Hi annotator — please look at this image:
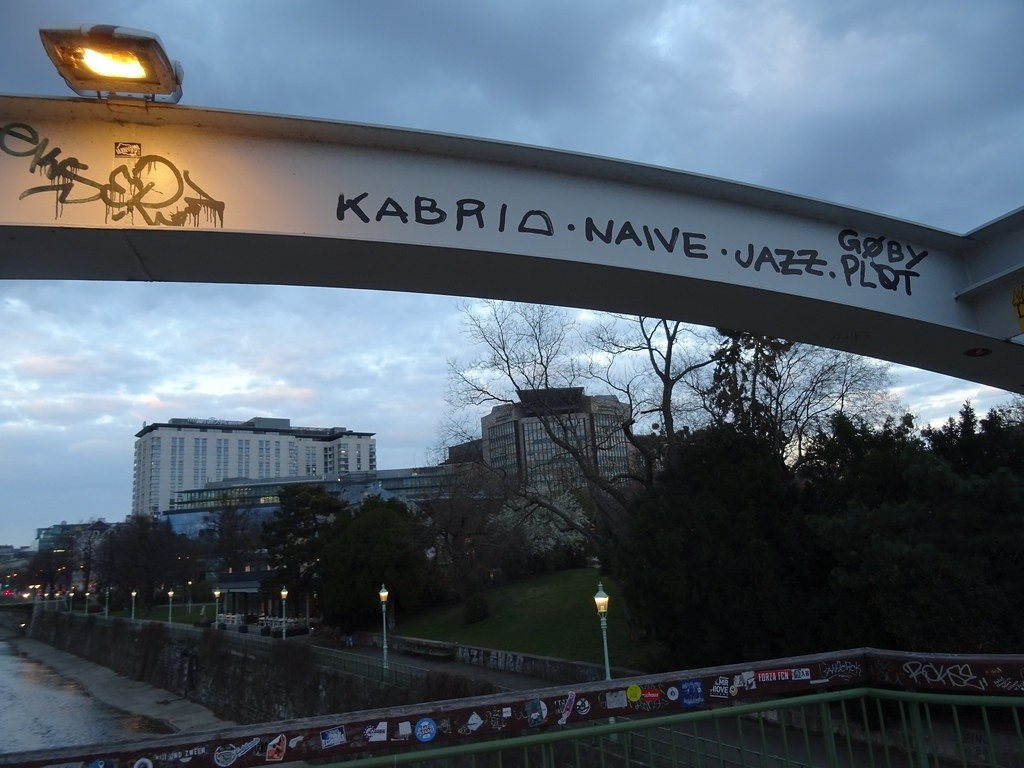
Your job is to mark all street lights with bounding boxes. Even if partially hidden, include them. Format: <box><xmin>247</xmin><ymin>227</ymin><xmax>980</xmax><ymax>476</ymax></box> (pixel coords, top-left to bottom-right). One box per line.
<box><xmin>280</xmin><ymin>585</ymin><xmax>288</xmax><ymax>640</ymax></box>
<box><xmin>105</xmin><ymin>591</ymin><xmax>109</xmax><ymax>617</ymax></box>
<box><xmin>168</xmin><ymin>588</ymin><xmax>175</xmax><ymax>624</ymax></box>
<box><xmin>187</xmin><ymin>579</ymin><xmax>192</xmax><ymax>614</ymax></box>
<box><xmin>68</xmin><ymin>592</ymin><xmax>74</xmax><ymax>613</ymax></box>
<box><xmin>84</xmin><ymin>592</ymin><xmax>90</xmax><ymax>614</ymax></box>
<box><xmin>378</xmin><ymin>583</ymin><xmax>389</xmax><ymax>668</ymax></box>
<box><xmin>213</xmin><ymin>586</ymin><xmax>220</xmax><ymax>629</ymax></box>
<box><xmin>594</xmin><ymin>581</ymin><xmax>611</xmax><ymax>680</ymax></box>
<box><xmin>131</xmin><ymin>589</ymin><xmax>137</xmax><ymax>620</ymax></box>
